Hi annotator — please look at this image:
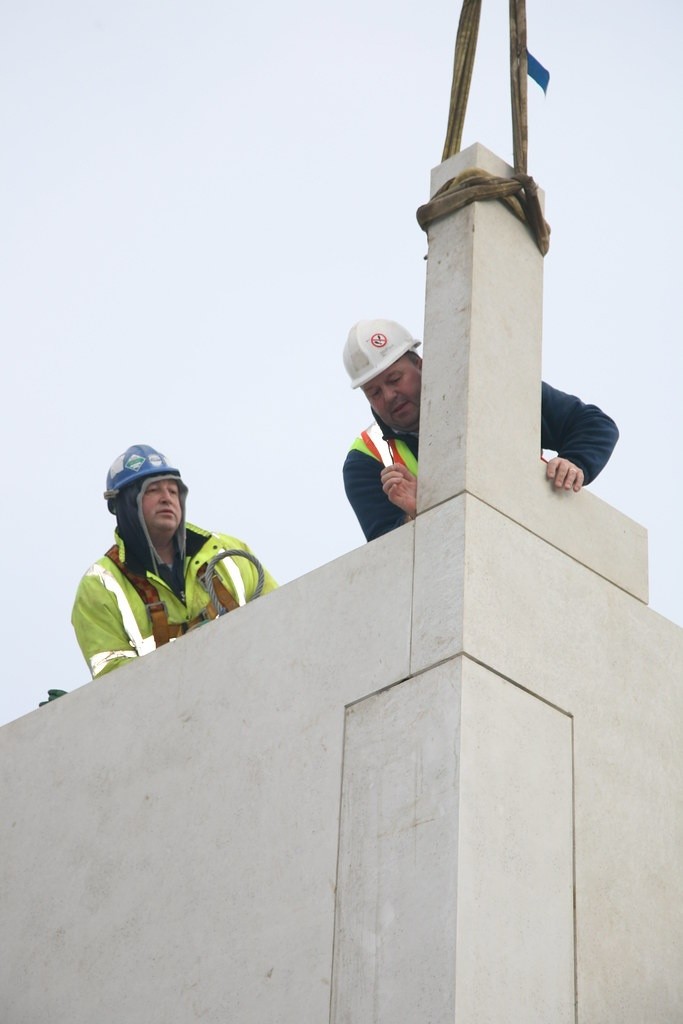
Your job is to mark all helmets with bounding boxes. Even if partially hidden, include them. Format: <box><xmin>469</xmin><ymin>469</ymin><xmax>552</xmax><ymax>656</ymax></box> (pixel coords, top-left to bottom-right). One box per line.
<box><xmin>102</xmin><ymin>444</ymin><xmax>180</xmax><ymax>513</ymax></box>
<box><xmin>341</xmin><ymin>318</ymin><xmax>422</xmax><ymax>389</ymax></box>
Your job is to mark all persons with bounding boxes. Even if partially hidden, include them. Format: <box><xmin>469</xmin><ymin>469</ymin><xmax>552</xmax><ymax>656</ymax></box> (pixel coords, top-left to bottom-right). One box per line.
<box><xmin>341</xmin><ymin>314</ymin><xmax>621</xmax><ymax>545</ymax></box>
<box><xmin>68</xmin><ymin>444</ymin><xmax>285</xmax><ymax>682</ymax></box>
